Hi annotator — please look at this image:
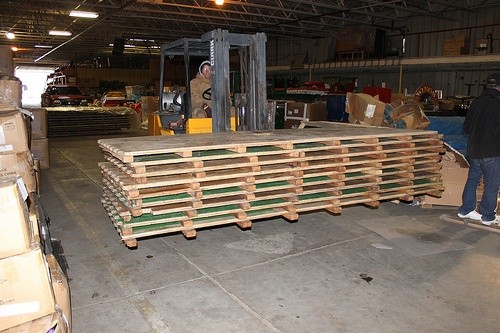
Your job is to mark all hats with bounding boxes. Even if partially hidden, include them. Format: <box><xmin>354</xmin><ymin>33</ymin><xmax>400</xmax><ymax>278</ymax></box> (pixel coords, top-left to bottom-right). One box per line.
<box><xmin>487</xmin><ymin>73</ymin><xmax>500</xmax><ymax>84</ymax></box>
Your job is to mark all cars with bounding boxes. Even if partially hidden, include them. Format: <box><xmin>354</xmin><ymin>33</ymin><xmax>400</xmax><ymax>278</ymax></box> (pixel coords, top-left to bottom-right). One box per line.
<box><xmin>101</xmin><ymin>92</ymin><xmax>144</xmax><ymax>119</ymax></box>
<box><xmin>41</xmin><ymin>84</ymin><xmax>86</xmax><ymax>109</ymax></box>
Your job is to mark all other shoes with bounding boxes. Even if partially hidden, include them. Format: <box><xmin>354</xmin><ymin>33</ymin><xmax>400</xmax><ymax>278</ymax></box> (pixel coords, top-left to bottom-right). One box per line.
<box><xmin>481</xmin><ymin>214</ymin><xmax>500</xmax><ymax>225</ymax></box>
<box><xmin>457</xmin><ymin>210</ymin><xmax>481</xmax><ymax>220</ymax></box>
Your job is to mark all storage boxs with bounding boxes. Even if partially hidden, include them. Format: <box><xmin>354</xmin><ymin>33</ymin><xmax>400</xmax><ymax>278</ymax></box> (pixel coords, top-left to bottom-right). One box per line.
<box><xmin>442</xmin><ymin>35</ymin><xmax>468</xmax><ymax>57</ymax></box>
<box><xmin>283</xmin><ymin>93</ymin><xmax>453</xmax><ymax>129</ymax></box>
<box><xmin>0</xmin><ymin>44</ymin><xmax>71</xmax><ymax>333</ymax></box>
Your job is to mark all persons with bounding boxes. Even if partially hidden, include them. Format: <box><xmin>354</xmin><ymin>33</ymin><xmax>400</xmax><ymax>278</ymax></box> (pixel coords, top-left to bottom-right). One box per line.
<box><xmin>189</xmin><ymin>60</ymin><xmax>212</xmax><ymax>118</ymax></box>
<box><xmin>457</xmin><ymin>72</ymin><xmax>500</xmax><ymax>225</ymax></box>
